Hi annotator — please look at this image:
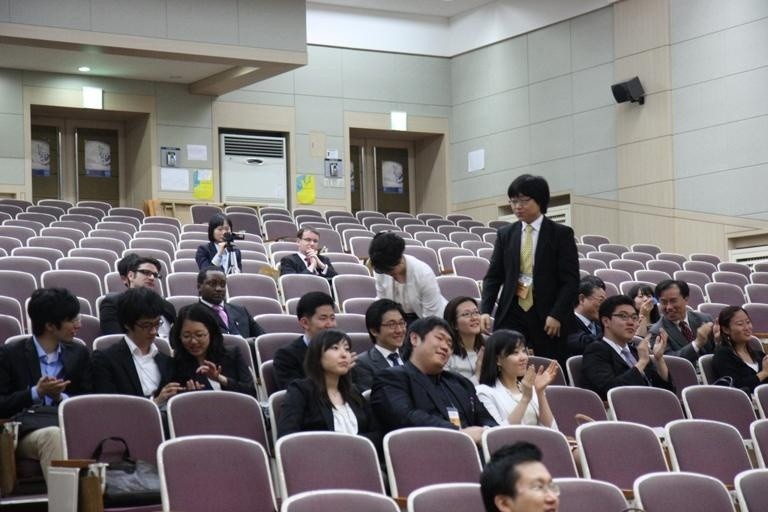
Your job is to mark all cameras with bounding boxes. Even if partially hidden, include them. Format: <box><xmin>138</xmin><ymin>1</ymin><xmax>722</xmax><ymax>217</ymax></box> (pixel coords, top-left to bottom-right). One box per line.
<box><xmin>223</xmin><ymin>232</ymin><xmax>245</xmax><ymax>247</ymax></box>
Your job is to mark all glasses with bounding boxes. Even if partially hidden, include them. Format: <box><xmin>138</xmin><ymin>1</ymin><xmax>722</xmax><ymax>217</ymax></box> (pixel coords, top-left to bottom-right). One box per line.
<box><xmin>463</xmin><ymin>309</ymin><xmax>481</xmax><ymax>318</ymax></box>
<box><xmin>522</xmin><ymin>479</ymin><xmax>561</xmax><ymax>498</ymax></box>
<box><xmin>136</xmin><ymin>269</ymin><xmax>158</xmax><ymax>278</ymax></box>
<box><xmin>135</xmin><ymin>319</ymin><xmax>163</xmax><ymax>331</ymax></box>
<box><xmin>611</xmin><ymin>312</ymin><xmax>639</xmax><ymax>321</ymax></box>
<box><xmin>591</xmin><ymin>295</ymin><xmax>603</xmax><ymax>304</ymax></box>
<box><xmin>181</xmin><ymin>331</ymin><xmax>207</xmax><ymax>340</ymax></box>
<box><xmin>380</xmin><ymin>320</ymin><xmax>407</xmax><ymax>329</ymax></box>
<box><xmin>507</xmin><ymin>197</ymin><xmax>532</xmax><ymax>207</ymax></box>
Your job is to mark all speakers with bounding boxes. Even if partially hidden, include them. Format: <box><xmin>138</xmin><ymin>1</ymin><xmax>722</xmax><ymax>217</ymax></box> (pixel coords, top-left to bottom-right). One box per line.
<box><xmin>610</xmin><ymin>75</ymin><xmax>644</xmax><ymax>103</ymax></box>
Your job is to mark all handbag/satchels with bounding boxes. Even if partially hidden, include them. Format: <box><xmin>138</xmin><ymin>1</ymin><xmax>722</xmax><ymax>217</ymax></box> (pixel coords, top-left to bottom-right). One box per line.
<box><xmin>11</xmin><ymin>405</ymin><xmax>58</xmax><ymax>435</ymax></box>
<box><xmin>713</xmin><ymin>376</ymin><xmax>752</xmax><ymax>401</ymax></box>
<box><xmin>567</xmin><ymin>414</ymin><xmax>596</xmax><ymax>473</ymax></box>
<box><xmin>80</xmin><ymin>437</ymin><xmax>161</xmax><ymax>505</ymax></box>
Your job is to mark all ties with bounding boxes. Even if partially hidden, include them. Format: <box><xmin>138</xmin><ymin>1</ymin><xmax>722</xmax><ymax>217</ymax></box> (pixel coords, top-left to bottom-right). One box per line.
<box><xmin>304</xmin><ymin>258</ymin><xmax>317</xmax><ymax>274</ymax></box>
<box><xmin>389</xmin><ymin>353</ymin><xmax>399</xmax><ymax>366</ymax></box>
<box><xmin>588</xmin><ymin>321</ymin><xmax>595</xmax><ymax>335</ymax></box>
<box><xmin>679</xmin><ymin>320</ymin><xmax>693</xmax><ymax>342</ymax></box>
<box><xmin>213</xmin><ymin>305</ymin><xmax>228</xmax><ymax>327</ymax></box>
<box><xmin>621</xmin><ymin>349</ymin><xmax>637</xmax><ymax>369</ymax></box>
<box><xmin>518</xmin><ymin>225</ymin><xmax>534</xmax><ymax>312</ymax></box>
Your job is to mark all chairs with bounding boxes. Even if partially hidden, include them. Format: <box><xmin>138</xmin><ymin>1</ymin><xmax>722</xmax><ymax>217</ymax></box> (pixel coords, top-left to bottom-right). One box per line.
<box><xmin>1</xmin><ymin>197</ymin><xmax>766</xmax><ymax>510</ymax></box>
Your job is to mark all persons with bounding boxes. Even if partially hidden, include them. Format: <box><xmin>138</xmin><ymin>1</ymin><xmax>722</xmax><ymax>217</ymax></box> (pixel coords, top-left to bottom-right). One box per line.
<box><xmin>555</xmin><ymin>273</ymin><xmax>767</xmax><ymax>421</ymax></box>
<box><xmin>480</xmin><ymin>439</ymin><xmax>561</xmax><ymax>511</ymax></box>
<box><xmin>479</xmin><ymin>173</ymin><xmax>579</xmax><ymax>352</ymax></box>
<box><xmin>193</xmin><ymin>213</ymin><xmax>245</xmax><ymax>275</ymax></box>
<box><xmin>278</xmin><ymin>224</ymin><xmax>338</xmax><ymax>276</ymax></box>
<box><xmin>1</xmin><ymin>252</ymin><xmax>558</xmax><ymax>496</ymax></box>
<box><xmin>369</xmin><ymin>232</ymin><xmax>450</xmax><ymax>323</ymax></box>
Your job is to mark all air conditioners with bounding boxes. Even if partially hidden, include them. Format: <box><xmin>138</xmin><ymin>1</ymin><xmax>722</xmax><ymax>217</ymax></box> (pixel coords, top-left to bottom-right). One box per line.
<box><xmin>219</xmin><ymin>133</ymin><xmax>289</xmax><ymax>207</ymax></box>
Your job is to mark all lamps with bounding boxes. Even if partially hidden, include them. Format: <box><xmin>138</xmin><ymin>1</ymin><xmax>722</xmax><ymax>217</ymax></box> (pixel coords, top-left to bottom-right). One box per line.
<box><xmin>610</xmin><ymin>76</ymin><xmax>647</xmax><ymax>108</ymax></box>
